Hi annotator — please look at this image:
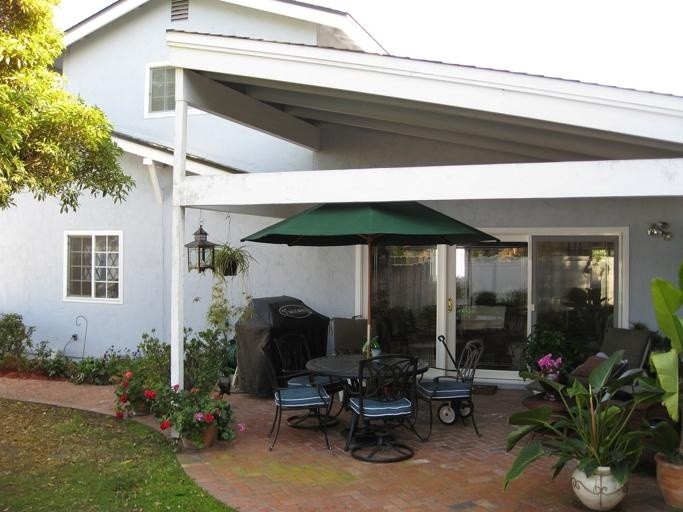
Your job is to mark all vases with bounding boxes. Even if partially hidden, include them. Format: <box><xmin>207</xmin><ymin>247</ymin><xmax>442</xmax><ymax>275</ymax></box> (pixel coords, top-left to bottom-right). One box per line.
<box><xmin>539</xmin><ymin>371</ymin><xmax>567</xmax><ymax>403</ymax></box>
<box><xmin>127</xmin><ymin>393</ymin><xmax>154</xmax><ymax>417</ymax></box>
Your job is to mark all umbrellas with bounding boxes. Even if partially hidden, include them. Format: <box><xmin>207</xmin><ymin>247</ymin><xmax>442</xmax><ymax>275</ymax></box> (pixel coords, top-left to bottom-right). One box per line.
<box><xmin>237</xmin><ymin>202</ymin><xmax>501</xmax><ymax>362</ymax></box>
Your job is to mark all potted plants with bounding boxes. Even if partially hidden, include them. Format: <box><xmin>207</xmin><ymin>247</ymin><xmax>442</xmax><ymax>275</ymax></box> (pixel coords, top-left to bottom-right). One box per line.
<box><xmin>208</xmin><ymin>241</ymin><xmax>257</xmax><ymax>278</ymax></box>
<box><xmin>636</xmin><ymin>263</ymin><xmax>683</xmax><ymax>509</ymax></box>
<box><xmin>180</xmin><ymin>424</ymin><xmax>218</xmax><ymax>450</ymax></box>
<box><xmin>501</xmin><ymin>350</ymin><xmax>678</xmax><ymax>511</ymax></box>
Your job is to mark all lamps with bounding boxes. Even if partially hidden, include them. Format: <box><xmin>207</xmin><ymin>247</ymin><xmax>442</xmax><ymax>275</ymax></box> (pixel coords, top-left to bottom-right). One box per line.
<box><xmin>183</xmin><ymin>208</ymin><xmax>218</xmax><ymax>274</ymax></box>
<box><xmin>646</xmin><ymin>220</ymin><xmax>675</xmax><ymax>244</ymax></box>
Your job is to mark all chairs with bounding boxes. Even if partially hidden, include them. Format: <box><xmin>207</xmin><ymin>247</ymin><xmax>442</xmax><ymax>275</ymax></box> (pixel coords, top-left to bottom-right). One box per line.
<box><xmin>525</xmin><ymin>326</ymin><xmax>651</xmax><ymax>391</ymax></box>
<box><xmin>257</xmin><ymin>314</ymin><xmax>487</xmax><ymax>462</ymax></box>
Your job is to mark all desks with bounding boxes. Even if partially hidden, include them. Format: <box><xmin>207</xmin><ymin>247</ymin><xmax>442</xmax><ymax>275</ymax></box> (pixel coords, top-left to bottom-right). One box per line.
<box><xmin>521</xmin><ymin>392</ymin><xmax>584</xmax><ymax>449</ymax></box>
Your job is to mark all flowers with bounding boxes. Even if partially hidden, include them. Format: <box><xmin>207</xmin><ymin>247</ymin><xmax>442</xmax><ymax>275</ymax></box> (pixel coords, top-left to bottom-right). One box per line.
<box><xmin>113</xmin><ymin>367</ymin><xmax>169</xmax><ymax>417</ymax></box>
<box><xmin>156</xmin><ymin>383</ymin><xmax>246</xmax><ymax>450</ymax></box>
<box><xmin>519</xmin><ymin>318</ymin><xmax>583</xmax><ymax>375</ymax></box>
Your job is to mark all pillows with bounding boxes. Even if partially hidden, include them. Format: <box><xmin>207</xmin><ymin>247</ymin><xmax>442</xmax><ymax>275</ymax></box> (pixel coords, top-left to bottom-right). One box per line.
<box><xmin>570</xmin><ymin>350</ymin><xmax>623</xmax><ymax>392</ymax></box>
